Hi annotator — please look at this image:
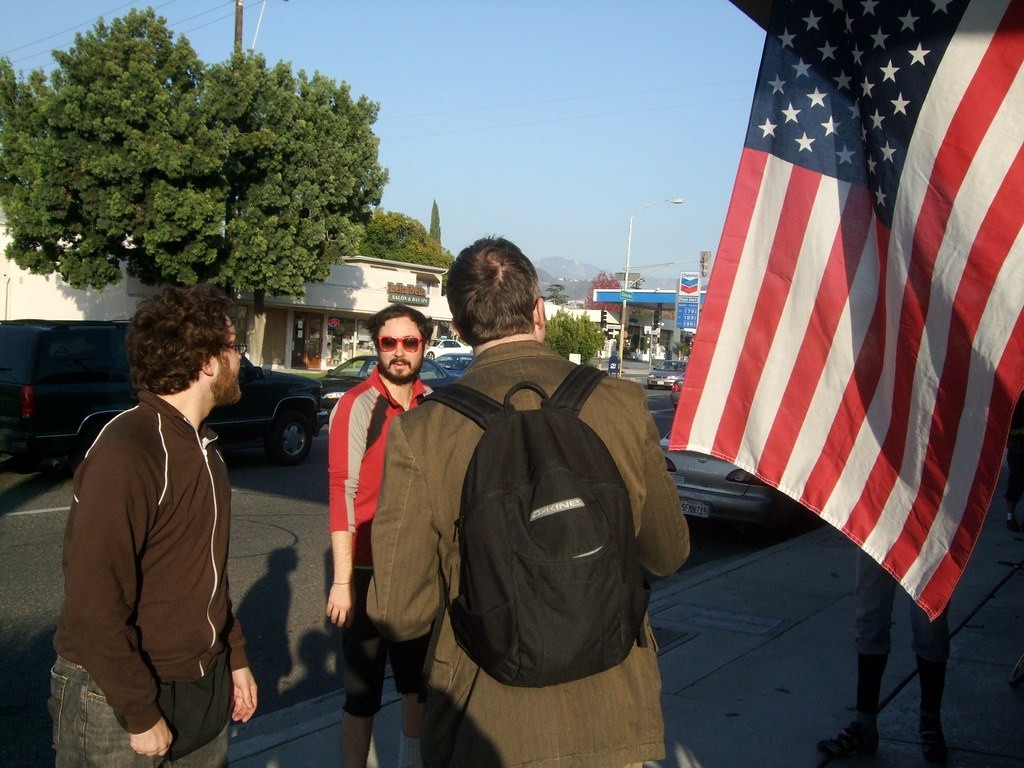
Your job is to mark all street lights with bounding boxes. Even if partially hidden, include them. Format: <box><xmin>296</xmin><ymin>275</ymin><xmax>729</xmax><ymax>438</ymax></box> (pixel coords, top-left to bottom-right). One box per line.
<box><xmin>615</xmin><ymin>198</ymin><xmax>685</xmax><ymax>378</ymax></box>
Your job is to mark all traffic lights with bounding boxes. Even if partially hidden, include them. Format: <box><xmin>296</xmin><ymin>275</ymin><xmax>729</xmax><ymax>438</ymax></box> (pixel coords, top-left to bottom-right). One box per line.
<box><xmin>652</xmin><ymin>311</ymin><xmax>660</xmax><ymax>330</ymax></box>
<box><xmin>601</xmin><ymin>311</ymin><xmax>607</xmax><ymax>325</ymax></box>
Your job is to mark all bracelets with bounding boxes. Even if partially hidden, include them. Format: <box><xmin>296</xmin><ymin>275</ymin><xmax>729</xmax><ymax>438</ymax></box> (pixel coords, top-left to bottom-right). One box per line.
<box><xmin>332</xmin><ymin>579</ymin><xmax>354</xmax><ymax>584</ymax></box>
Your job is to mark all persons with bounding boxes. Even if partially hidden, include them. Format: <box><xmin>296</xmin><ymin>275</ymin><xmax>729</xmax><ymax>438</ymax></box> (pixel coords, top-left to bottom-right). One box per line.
<box><xmin>325</xmin><ymin>304</ymin><xmax>435</xmax><ymax>768</ymax></box>
<box><xmin>1005</xmin><ymin>391</ymin><xmax>1024</xmax><ymax>530</ymax></box>
<box><xmin>367</xmin><ymin>237</ymin><xmax>690</xmax><ymax>768</ymax></box>
<box><xmin>326</xmin><ymin>342</ymin><xmax>342</xmax><ymax>366</ymax></box>
<box><xmin>816</xmin><ymin>545</ymin><xmax>950</xmax><ymax>763</ymax></box>
<box><xmin>49</xmin><ymin>285</ymin><xmax>257</xmax><ymax>768</ymax></box>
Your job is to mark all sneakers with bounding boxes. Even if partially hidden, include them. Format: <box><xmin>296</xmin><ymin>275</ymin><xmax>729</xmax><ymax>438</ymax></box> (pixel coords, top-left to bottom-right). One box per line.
<box><xmin>817</xmin><ymin>728</ymin><xmax>879</xmax><ymax>757</ymax></box>
<box><xmin>919</xmin><ymin>717</ymin><xmax>945</xmax><ymax>762</ymax></box>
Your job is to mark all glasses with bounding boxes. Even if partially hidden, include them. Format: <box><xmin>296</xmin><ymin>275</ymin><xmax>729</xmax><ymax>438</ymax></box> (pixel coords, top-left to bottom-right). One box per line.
<box><xmin>378</xmin><ymin>335</ymin><xmax>422</xmax><ymax>352</ymax></box>
<box><xmin>220</xmin><ymin>344</ymin><xmax>247</xmax><ymax>354</ymax></box>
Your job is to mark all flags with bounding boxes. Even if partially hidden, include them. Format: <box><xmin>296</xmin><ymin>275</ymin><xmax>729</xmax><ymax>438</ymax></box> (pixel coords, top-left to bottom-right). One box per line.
<box><xmin>671</xmin><ymin>1</ymin><xmax>1024</xmax><ymax>617</ymax></box>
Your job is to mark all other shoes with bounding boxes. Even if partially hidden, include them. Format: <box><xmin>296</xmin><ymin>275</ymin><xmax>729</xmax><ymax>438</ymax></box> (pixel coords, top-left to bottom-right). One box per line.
<box><xmin>1007</xmin><ymin>519</ymin><xmax>1021</xmax><ymax>532</ymax></box>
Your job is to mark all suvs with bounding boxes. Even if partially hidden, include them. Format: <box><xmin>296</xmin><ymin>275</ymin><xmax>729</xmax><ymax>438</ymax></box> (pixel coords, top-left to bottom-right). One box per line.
<box><xmin>0</xmin><ymin>318</ymin><xmax>331</xmax><ymax>465</ymax></box>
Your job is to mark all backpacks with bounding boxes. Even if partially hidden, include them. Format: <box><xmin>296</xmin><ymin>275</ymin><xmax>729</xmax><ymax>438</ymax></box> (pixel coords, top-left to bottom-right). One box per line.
<box><xmin>418</xmin><ymin>364</ymin><xmax>652</xmax><ymax>687</ymax></box>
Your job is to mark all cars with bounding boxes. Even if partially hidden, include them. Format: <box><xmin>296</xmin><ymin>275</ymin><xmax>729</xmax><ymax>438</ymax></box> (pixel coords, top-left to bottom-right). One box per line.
<box><xmin>426</xmin><ymin>338</ymin><xmax>475</xmax><ymax>363</ymax></box>
<box><xmin>310</xmin><ymin>356</ymin><xmax>463</xmax><ymax>427</ymax></box>
<box><xmin>659</xmin><ymin>429</ymin><xmax>827</xmax><ymax>535</ymax></box>
<box><xmin>436</xmin><ymin>353</ymin><xmax>476</xmax><ymax>377</ymax></box>
<box><xmin>647</xmin><ymin>360</ymin><xmax>688</xmax><ymax>388</ymax></box>
